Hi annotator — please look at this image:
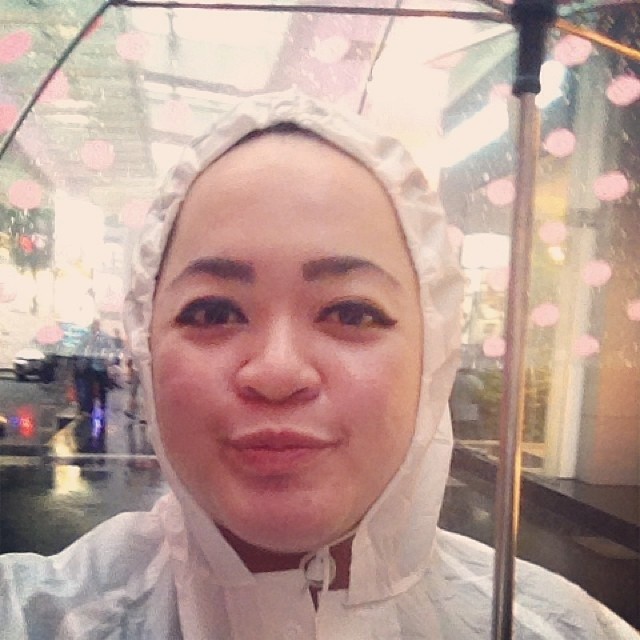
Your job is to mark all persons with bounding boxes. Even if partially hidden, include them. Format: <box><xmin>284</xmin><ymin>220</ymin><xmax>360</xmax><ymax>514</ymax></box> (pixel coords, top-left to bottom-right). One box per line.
<box><xmin>76</xmin><ymin>320</ymin><xmax>117</xmax><ymax>419</ymax></box>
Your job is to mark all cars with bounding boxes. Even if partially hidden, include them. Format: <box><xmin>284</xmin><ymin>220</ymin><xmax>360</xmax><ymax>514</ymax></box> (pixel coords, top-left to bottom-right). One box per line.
<box><xmin>13</xmin><ymin>322</ymin><xmax>117</xmax><ymax>381</ymax></box>
